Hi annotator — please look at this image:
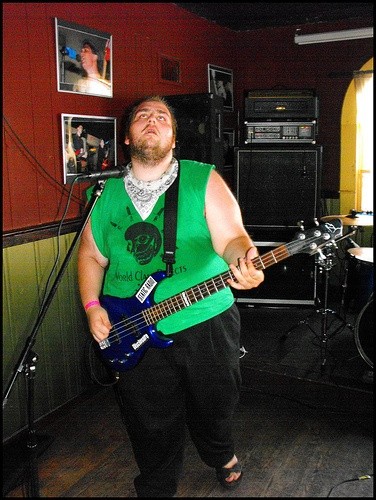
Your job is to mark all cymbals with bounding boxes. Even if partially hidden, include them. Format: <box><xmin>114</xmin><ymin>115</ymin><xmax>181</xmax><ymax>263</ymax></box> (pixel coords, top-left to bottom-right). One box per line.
<box><xmin>347</xmin><ymin>243</ymin><xmax>375</xmax><ymax>266</ymax></box>
<box><xmin>320</xmin><ymin>210</ymin><xmax>374</xmax><ymax>229</ymax></box>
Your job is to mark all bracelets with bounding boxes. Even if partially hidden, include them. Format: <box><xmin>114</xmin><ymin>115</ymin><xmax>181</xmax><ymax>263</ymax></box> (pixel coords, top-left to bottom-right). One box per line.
<box><xmin>84</xmin><ymin>300</ymin><xmax>99</xmax><ymax>311</ymax></box>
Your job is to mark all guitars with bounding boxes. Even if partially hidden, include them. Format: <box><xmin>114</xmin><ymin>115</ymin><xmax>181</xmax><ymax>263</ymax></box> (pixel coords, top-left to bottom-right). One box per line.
<box><xmin>91</xmin><ymin>215</ymin><xmax>345</xmax><ymax>373</ymax></box>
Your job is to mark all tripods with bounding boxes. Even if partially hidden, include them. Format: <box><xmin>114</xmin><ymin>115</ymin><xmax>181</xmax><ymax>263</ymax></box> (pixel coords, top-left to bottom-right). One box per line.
<box><xmin>278</xmin><ymin>208</ymin><xmax>374</xmax><ymax>374</ymax></box>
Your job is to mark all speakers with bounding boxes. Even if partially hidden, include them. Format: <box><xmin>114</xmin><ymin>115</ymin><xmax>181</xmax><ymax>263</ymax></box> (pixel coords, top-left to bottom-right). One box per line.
<box><xmin>232</xmin><ymin>144</ymin><xmax>323</xmax><ymax>233</ymax></box>
<box><xmin>164</xmin><ymin>93</ymin><xmax>224</xmax><ymax>175</ymax></box>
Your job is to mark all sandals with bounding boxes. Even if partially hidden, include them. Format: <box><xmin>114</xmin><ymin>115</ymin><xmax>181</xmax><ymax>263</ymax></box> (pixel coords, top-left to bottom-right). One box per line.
<box><xmin>216</xmin><ymin>454</ymin><xmax>243</xmax><ymax>490</ymax></box>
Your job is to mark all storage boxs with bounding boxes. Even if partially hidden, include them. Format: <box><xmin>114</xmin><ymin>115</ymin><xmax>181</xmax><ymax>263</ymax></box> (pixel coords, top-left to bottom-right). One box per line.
<box><xmin>233</xmin><ymin>241</ymin><xmax>318</xmax><ymax>307</ymax></box>
<box><xmin>233</xmin><ymin>144</ymin><xmax>323</xmax><ymax>228</ymax></box>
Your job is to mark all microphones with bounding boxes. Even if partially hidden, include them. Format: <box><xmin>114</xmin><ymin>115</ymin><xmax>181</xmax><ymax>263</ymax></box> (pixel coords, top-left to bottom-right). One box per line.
<box><xmin>76</xmin><ymin>165</ymin><xmax>128</xmax><ymax>182</ymax></box>
<box><xmin>61</xmin><ymin>47</ymin><xmax>81</xmax><ymax>62</ymax></box>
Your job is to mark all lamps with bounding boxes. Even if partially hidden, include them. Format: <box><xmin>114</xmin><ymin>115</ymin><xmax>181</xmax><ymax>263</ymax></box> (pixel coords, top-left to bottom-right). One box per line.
<box><xmin>294</xmin><ymin>25</ymin><xmax>373</xmax><ymax>45</ymax></box>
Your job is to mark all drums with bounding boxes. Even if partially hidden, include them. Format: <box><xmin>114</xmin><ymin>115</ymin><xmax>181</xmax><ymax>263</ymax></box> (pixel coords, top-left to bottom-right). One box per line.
<box><xmin>353</xmin><ymin>290</ymin><xmax>376</xmax><ymax>369</ymax></box>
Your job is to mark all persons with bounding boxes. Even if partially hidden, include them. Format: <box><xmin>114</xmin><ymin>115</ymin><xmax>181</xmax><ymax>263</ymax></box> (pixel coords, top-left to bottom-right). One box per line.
<box><xmin>72</xmin><ymin>38</ymin><xmax>113</xmax><ymax>98</ymax></box>
<box><xmin>77</xmin><ymin>96</ymin><xmax>265</xmax><ymax>500</ymax></box>
<box><xmin>66</xmin><ymin>117</ymin><xmax>112</xmax><ymax>173</ymax></box>
